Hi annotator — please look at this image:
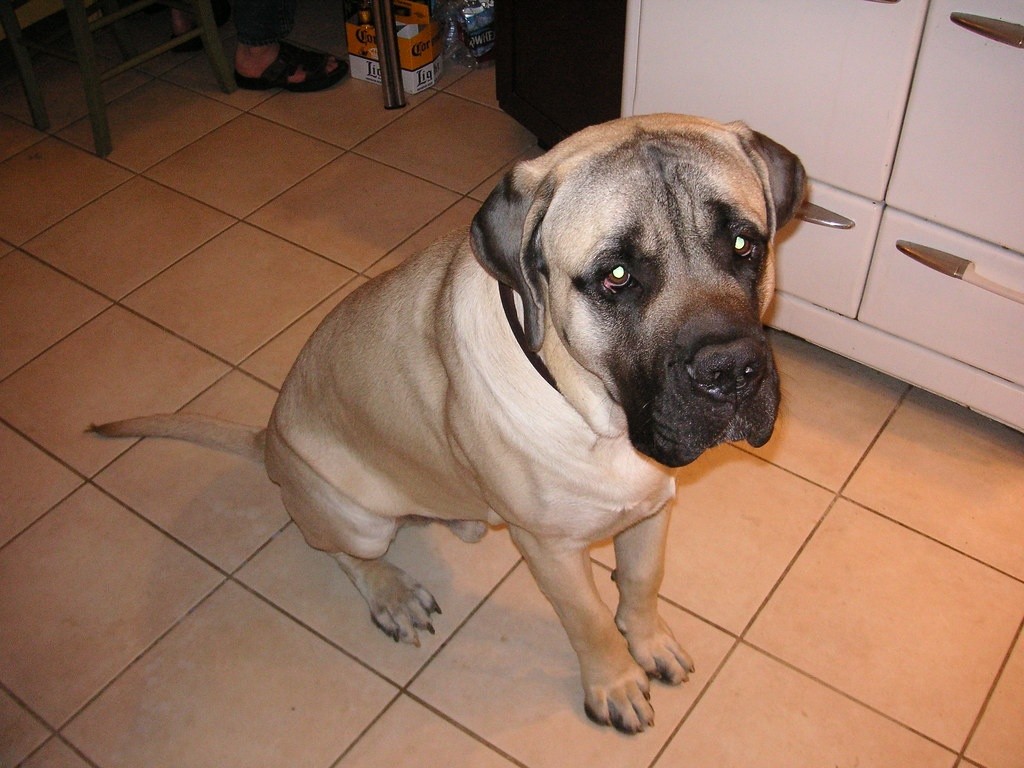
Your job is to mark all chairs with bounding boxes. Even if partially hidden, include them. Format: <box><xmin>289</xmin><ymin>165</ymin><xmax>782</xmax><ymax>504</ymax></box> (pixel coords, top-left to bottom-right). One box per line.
<box><xmin>0</xmin><ymin>0</ymin><xmax>237</xmax><ymax>158</ymax></box>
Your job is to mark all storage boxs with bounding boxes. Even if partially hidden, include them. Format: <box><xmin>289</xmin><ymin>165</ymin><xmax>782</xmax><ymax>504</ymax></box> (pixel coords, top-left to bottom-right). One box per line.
<box><xmin>346</xmin><ymin>0</ymin><xmax>443</xmax><ymax>94</ymax></box>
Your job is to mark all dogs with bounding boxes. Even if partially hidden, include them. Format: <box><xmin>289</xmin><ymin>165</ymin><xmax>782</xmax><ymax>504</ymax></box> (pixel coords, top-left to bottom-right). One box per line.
<box><xmin>82</xmin><ymin>113</ymin><xmax>808</xmax><ymax>735</ymax></box>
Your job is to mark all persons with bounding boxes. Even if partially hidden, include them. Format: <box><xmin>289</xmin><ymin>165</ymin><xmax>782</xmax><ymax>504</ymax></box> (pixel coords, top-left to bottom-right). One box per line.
<box><xmin>168</xmin><ymin>0</ymin><xmax>349</xmax><ymax>93</ymax></box>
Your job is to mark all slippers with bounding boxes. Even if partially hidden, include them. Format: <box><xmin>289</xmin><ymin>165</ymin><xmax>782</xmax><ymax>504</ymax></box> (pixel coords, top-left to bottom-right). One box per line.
<box><xmin>171</xmin><ymin>0</ymin><xmax>231</xmax><ymax>54</ymax></box>
<box><xmin>234</xmin><ymin>42</ymin><xmax>349</xmax><ymax>91</ymax></box>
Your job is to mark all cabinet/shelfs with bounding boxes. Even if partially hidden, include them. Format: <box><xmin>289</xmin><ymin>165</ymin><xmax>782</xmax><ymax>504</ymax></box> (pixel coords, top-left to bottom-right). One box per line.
<box><xmin>622</xmin><ymin>0</ymin><xmax>1024</xmax><ymax>434</ymax></box>
